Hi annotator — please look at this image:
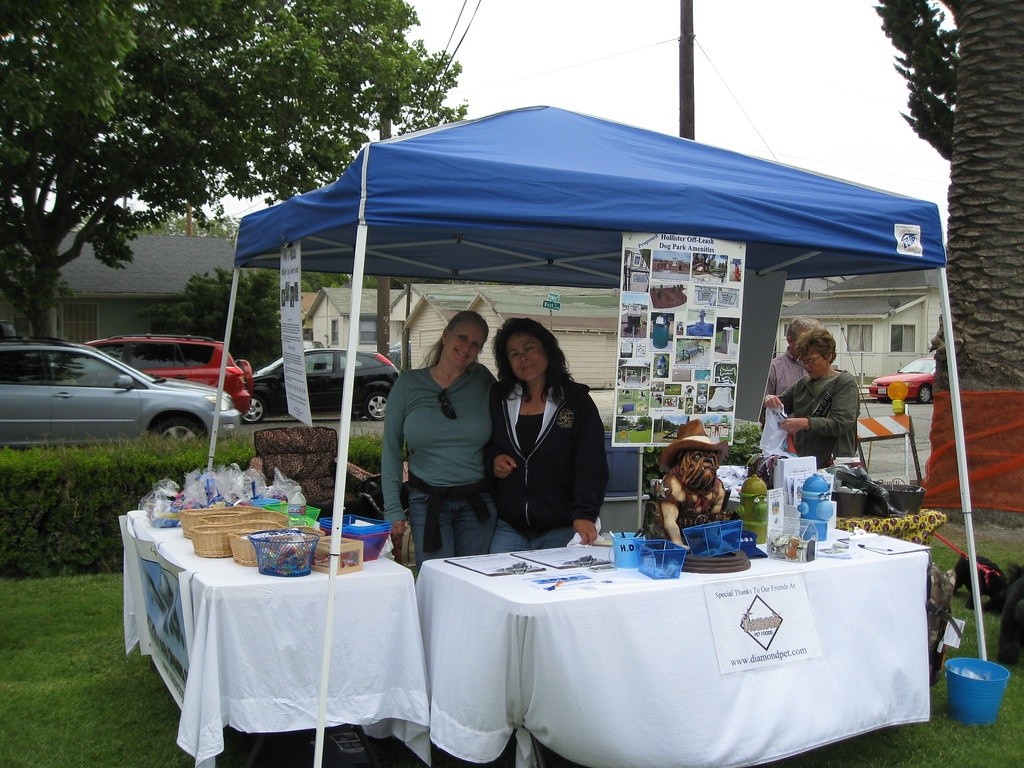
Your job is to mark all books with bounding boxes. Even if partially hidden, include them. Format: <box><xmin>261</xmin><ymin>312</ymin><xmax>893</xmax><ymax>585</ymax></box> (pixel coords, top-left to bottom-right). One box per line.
<box><xmin>774</xmin><ymin>455</ymin><xmax>835</xmax><ymax>506</ymax></box>
<box><xmin>766</xmin><ymin>488</ymin><xmax>783</xmax><ymax>534</ymax></box>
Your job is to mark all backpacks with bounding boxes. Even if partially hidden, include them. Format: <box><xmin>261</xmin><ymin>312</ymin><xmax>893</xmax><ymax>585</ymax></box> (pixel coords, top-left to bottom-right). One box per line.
<box><xmin>926</xmin><ymin>567</ymin><xmax>958</xmax><ymax>684</ymax></box>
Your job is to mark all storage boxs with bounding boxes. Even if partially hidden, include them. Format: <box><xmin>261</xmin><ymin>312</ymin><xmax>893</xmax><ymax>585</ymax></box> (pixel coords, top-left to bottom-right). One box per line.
<box><xmin>634</xmin><ymin>539</ymin><xmax>687</xmax><ymax>580</ymax></box>
<box><xmin>681</xmin><ymin>519</ymin><xmax>743</xmax><ymax>557</ymax></box>
<box><xmin>768</xmin><ymin>517</ymin><xmax>819</xmax><ymax>563</ymax></box>
<box><xmin>264</xmin><ymin>503</ymin><xmax>391</xmax><ymax>574</ymax></box>
<box><xmin>773</xmin><ymin>456</ymin><xmax>817</xmax><ymax>492</ymax></box>
<box><xmin>605</xmin><ymin>431</ymin><xmax>644</xmax><ymax>497</ymax></box>
<box><xmin>783</xmin><ymin>501</ymin><xmax>838</xmax><ymax>536</ymax></box>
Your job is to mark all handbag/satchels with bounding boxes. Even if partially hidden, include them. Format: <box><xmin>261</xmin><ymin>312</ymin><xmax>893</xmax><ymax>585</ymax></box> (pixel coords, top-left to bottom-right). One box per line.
<box><xmin>826</xmin><ymin>457</ymin><xmax>865</xmax><ymax>471</ymax></box>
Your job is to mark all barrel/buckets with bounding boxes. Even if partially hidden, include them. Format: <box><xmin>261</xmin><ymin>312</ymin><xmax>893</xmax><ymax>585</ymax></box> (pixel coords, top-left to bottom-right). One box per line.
<box><xmin>832</xmin><ymin>492</ymin><xmax>868</xmax><ymax>518</ymax></box>
<box><xmin>653</xmin><ymin>321</ymin><xmax>669</xmax><ymax>349</ymax></box>
<box><xmin>881</xmin><ymin>485</ymin><xmax>926</xmax><ymax>515</ymax></box>
<box><xmin>945</xmin><ymin>658</ymin><xmax>1010</xmax><ymax>725</ymax></box>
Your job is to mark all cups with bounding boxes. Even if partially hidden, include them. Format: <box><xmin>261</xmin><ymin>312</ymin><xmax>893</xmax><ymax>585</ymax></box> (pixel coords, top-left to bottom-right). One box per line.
<box><xmin>612</xmin><ymin>533</ymin><xmax>644</xmax><ymax>568</ymax></box>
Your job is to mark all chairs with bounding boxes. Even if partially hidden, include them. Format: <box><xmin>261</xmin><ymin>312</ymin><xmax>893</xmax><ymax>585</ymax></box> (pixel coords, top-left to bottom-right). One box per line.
<box><xmin>254</xmin><ymin>426</ymin><xmax>338</xmax><ymax>522</ymax></box>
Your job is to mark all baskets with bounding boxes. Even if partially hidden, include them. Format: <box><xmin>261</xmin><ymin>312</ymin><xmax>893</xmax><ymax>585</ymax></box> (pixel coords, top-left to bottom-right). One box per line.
<box><xmin>183</xmin><ymin>498</ymin><xmax>391</xmax><ymax>581</ymax></box>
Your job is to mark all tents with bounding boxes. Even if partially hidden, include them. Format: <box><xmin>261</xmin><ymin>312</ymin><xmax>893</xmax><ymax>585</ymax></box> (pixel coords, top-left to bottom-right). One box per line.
<box><xmin>206</xmin><ymin>105</ymin><xmax>987</xmax><ymax>768</ymax></box>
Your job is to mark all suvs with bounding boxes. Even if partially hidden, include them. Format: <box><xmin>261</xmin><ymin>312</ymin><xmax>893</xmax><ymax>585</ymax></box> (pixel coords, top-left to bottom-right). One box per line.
<box><xmin>241</xmin><ymin>348</ymin><xmax>401</xmax><ymax>424</ymax></box>
<box><xmin>0</xmin><ymin>337</ymin><xmax>242</xmax><ymax>454</ymax></box>
<box><xmin>63</xmin><ymin>332</ymin><xmax>255</xmax><ymax>418</ymax></box>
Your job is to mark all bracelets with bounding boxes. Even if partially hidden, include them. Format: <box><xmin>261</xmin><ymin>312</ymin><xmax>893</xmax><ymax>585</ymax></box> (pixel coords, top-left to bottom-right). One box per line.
<box><xmin>804</xmin><ymin>416</ymin><xmax>812</xmax><ymax>432</ymax></box>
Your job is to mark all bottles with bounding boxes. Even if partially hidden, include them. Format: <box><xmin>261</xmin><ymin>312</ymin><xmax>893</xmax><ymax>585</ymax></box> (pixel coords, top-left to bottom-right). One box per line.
<box><xmin>737</xmin><ymin>474</ymin><xmax>768</xmax><ymax>544</ymax></box>
<box><xmin>798</xmin><ymin>474</ymin><xmax>833</xmax><ymax>541</ymax></box>
<box><xmin>288</xmin><ymin>486</ymin><xmax>306</xmax><ymax>527</ymax></box>
<box><xmin>654</xmin><ymin>258</ymin><xmax>689</xmax><ymax>274</ymax></box>
<box><xmin>657</xmin><ymin>356</ymin><xmax>665</xmax><ymax>376</ymax></box>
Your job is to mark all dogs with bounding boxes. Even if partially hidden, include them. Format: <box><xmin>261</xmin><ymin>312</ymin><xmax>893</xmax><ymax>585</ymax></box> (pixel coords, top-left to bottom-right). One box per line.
<box><xmin>951</xmin><ymin>555</ymin><xmax>1007</xmax><ymax>611</ymax></box>
<box><xmin>983</xmin><ymin>563</ymin><xmax>1024</xmax><ymax>666</ymax></box>
<box><xmin>644</xmin><ymin>448</ymin><xmax>726</xmax><ymax>555</ymax></box>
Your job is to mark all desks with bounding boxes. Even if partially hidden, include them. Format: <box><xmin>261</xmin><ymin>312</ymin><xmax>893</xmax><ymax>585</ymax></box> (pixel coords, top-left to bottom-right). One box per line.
<box><xmin>836</xmin><ymin>508</ymin><xmax>948</xmax><ymax>546</ymax></box>
<box><xmin>416</xmin><ymin>529</ymin><xmax>932</xmax><ymax>768</ymax></box>
<box><xmin>117</xmin><ymin>510</ymin><xmax>430</xmax><ymax>768</ymax></box>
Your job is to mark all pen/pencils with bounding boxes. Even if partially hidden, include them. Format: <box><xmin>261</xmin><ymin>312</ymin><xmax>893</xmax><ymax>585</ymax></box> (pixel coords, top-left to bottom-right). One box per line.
<box><xmin>620</xmin><ymin>529</ymin><xmax>627</xmax><ymax>539</ymax></box>
<box><xmin>640</xmin><ymin>525</ymin><xmax>651</xmax><ymax>539</ymax></box>
<box><xmin>608</xmin><ymin>530</ymin><xmax>615</xmax><ymax>538</ymax></box>
<box><xmin>634</xmin><ymin>528</ymin><xmax>643</xmax><ymax>538</ymax></box>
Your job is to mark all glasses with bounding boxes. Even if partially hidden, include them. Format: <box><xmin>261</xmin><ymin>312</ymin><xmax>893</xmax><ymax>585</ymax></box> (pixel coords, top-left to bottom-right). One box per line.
<box><xmin>796</xmin><ymin>352</ymin><xmax>824</xmax><ymax>367</ymax></box>
<box><xmin>438</xmin><ymin>388</ymin><xmax>457</xmax><ymax>419</ymax></box>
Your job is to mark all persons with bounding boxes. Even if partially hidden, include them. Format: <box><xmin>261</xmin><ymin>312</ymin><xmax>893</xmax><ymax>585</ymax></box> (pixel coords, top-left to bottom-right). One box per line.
<box><xmin>734</xmin><ymin>264</ymin><xmax>739</xmax><ymax>281</ymax></box>
<box><xmin>765</xmin><ymin>328</ymin><xmax>860</xmax><ymax>466</ymax></box>
<box><xmin>54</xmin><ymin>356</ymin><xmax>78</xmax><ymax>383</ymax></box>
<box><xmin>763</xmin><ymin>315</ymin><xmax>821</xmax><ymax>448</ymax></box>
<box><xmin>484</xmin><ymin>318</ymin><xmax>609</xmax><ymax>555</ymax></box>
<box><xmin>381</xmin><ymin>311</ymin><xmax>499</xmax><ymax>574</ymax></box>
<box><xmin>652</xmin><ymin>383</ymin><xmax>663</xmax><ymax>391</ymax></box>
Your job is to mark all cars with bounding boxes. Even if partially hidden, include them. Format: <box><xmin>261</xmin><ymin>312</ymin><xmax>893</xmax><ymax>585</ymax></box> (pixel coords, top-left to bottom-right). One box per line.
<box><xmin>301</xmin><ymin>340</ymin><xmax>326</xmax><ymax>364</ymax></box>
<box><xmin>868</xmin><ymin>357</ymin><xmax>936</xmax><ymax>403</ymax></box>
<box><xmin>371</xmin><ymin>340</ymin><xmax>411</xmax><ymax>365</ymax></box>
